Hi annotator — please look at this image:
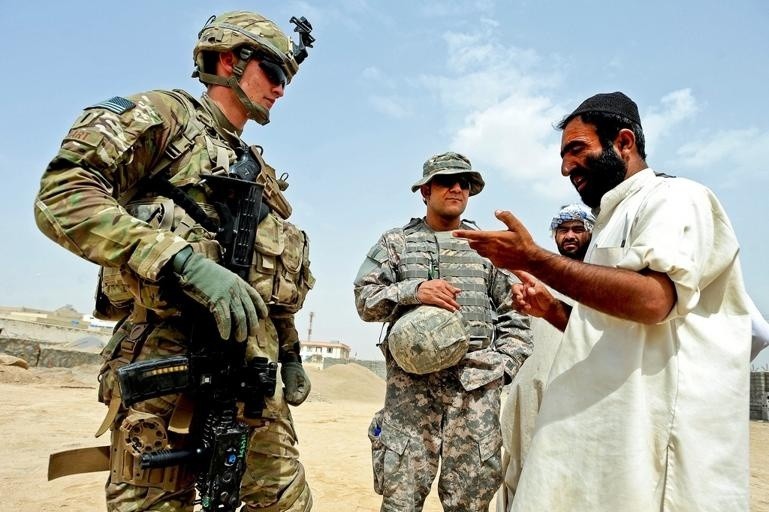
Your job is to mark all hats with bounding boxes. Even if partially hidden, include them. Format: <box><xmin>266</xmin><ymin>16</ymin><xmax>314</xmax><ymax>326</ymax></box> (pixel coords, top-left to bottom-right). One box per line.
<box><xmin>568</xmin><ymin>91</ymin><xmax>643</xmax><ymax>129</ymax></box>
<box><xmin>259</xmin><ymin>60</ymin><xmax>286</xmax><ymax>89</ymax></box>
<box><xmin>412</xmin><ymin>153</ymin><xmax>485</xmax><ymax>197</ymax></box>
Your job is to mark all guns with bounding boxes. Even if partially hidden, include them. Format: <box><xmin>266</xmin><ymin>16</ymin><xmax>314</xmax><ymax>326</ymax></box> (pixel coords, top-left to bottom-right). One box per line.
<box><xmin>116</xmin><ymin>175</ymin><xmax>277</xmax><ymax>512</ymax></box>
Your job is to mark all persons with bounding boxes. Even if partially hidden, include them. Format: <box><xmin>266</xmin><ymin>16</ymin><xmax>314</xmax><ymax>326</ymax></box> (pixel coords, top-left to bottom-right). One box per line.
<box><xmin>35</xmin><ymin>11</ymin><xmax>318</xmax><ymax>508</ymax></box>
<box><xmin>452</xmin><ymin>88</ymin><xmax>769</xmax><ymax>512</ymax></box>
<box><xmin>547</xmin><ymin>202</ymin><xmax>599</xmax><ymax>262</ymax></box>
<box><xmin>354</xmin><ymin>149</ymin><xmax>534</xmax><ymax>512</ymax></box>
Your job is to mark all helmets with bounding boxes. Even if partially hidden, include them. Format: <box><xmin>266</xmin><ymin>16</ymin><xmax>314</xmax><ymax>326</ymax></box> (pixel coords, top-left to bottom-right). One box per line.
<box><xmin>192</xmin><ymin>11</ymin><xmax>299</xmax><ymax>126</ymax></box>
<box><xmin>386</xmin><ymin>305</ymin><xmax>469</xmax><ymax>377</ymax></box>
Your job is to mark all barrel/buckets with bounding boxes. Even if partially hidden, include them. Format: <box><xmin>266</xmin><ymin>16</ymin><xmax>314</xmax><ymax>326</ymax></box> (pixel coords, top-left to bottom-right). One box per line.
<box><xmin>750</xmin><ymin>370</ymin><xmax>765</xmax><ymax>422</ymax></box>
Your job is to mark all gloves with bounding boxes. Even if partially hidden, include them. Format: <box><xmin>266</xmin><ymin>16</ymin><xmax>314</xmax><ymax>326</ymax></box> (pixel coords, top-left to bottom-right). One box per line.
<box><xmin>280</xmin><ymin>362</ymin><xmax>312</xmax><ymax>406</ymax></box>
<box><xmin>174</xmin><ymin>253</ymin><xmax>268</xmax><ymax>342</ymax></box>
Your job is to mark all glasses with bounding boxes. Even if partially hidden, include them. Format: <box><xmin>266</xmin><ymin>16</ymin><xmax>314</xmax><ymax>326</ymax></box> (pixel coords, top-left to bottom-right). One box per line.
<box><xmin>436</xmin><ymin>178</ymin><xmax>469</xmax><ymax>190</ymax></box>
